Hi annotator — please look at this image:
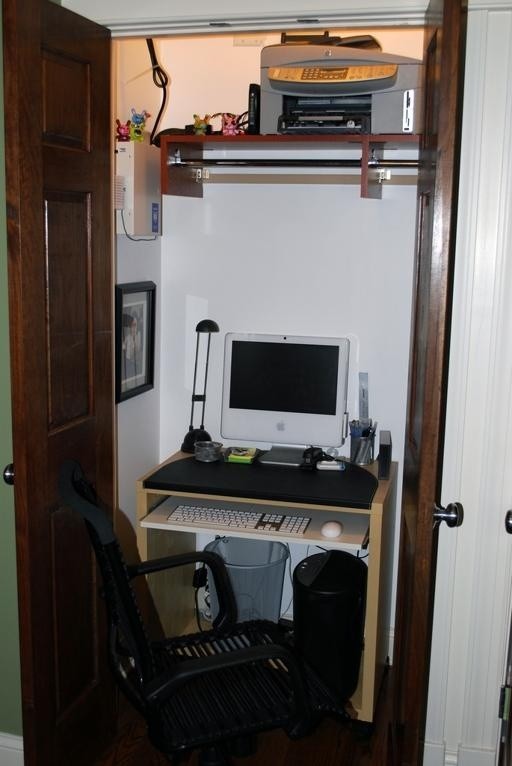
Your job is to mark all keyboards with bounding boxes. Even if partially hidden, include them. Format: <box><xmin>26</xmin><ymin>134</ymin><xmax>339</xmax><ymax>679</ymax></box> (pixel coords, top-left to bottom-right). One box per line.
<box><xmin>167</xmin><ymin>504</ymin><xmax>312</xmax><ymax>535</ymax></box>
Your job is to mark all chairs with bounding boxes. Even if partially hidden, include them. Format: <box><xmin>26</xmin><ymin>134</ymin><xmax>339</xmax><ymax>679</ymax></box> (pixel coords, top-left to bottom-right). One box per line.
<box><xmin>69</xmin><ymin>470</ymin><xmax>358</xmax><ymax>766</ymax></box>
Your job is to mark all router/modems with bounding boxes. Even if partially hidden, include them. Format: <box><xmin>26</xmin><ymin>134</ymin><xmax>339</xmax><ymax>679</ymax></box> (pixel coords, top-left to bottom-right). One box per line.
<box><xmin>247</xmin><ymin>83</ymin><xmax>260</xmax><ymax>135</ymax></box>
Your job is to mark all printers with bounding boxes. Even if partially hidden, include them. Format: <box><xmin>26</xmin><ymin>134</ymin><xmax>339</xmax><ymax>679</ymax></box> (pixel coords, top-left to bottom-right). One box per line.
<box><xmin>257</xmin><ymin>29</ymin><xmax>422</xmax><ymax>135</ymax></box>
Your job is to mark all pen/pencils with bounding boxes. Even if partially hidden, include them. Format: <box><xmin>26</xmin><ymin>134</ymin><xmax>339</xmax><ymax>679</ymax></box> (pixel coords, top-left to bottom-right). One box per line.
<box><xmin>350</xmin><ymin>419</ymin><xmax>377</xmax><ymax>463</ymax></box>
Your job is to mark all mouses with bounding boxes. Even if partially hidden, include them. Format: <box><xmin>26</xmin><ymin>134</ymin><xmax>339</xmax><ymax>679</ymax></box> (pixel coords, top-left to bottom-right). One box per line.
<box><xmin>321</xmin><ymin>520</ymin><xmax>343</xmax><ymax>537</ymax></box>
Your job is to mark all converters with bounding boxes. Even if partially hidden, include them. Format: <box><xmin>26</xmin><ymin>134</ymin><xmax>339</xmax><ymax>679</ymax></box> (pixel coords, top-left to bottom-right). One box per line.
<box><xmin>193</xmin><ymin>567</ymin><xmax>208</xmax><ymax>588</ymax></box>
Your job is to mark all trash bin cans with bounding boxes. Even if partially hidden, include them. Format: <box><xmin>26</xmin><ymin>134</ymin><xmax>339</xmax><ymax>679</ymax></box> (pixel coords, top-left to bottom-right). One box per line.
<box><xmin>204</xmin><ymin>535</ymin><xmax>289</xmax><ymax>629</ymax></box>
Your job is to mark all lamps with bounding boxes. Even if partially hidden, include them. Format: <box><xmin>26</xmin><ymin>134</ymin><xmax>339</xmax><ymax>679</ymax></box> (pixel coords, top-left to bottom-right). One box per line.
<box><xmin>182</xmin><ymin>320</ymin><xmax>220</xmax><ymax>452</ymax></box>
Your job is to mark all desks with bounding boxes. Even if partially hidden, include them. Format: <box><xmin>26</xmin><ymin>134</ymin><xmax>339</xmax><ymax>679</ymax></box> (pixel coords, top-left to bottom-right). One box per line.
<box><xmin>136</xmin><ymin>451</ymin><xmax>398</xmax><ymax>723</ymax></box>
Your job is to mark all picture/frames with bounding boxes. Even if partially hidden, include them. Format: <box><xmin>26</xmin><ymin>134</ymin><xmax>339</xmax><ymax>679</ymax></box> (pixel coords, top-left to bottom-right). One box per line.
<box><xmin>116</xmin><ymin>281</ymin><xmax>156</xmax><ymax>404</ymax></box>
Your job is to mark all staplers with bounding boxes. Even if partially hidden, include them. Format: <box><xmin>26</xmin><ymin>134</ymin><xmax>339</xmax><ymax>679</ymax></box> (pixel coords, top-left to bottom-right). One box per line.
<box><xmin>299</xmin><ymin>447</ymin><xmax>323</xmax><ymax>473</ymax></box>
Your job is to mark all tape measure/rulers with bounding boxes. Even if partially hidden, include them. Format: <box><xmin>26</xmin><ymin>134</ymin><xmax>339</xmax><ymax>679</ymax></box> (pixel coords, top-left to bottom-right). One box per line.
<box><xmin>360</xmin><ymin>373</ymin><xmax>369</xmax><ymax>420</ymax></box>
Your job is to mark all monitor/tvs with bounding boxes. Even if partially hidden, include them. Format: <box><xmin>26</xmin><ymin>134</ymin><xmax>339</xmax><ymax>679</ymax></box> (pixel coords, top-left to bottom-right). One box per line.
<box><xmin>219</xmin><ymin>331</ymin><xmax>352</xmax><ymax>466</ymax></box>
